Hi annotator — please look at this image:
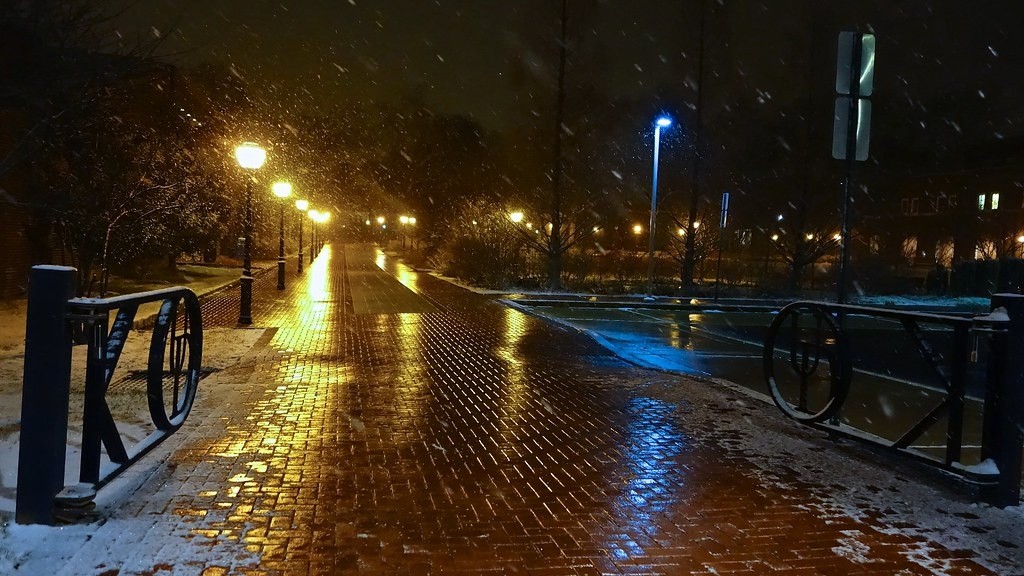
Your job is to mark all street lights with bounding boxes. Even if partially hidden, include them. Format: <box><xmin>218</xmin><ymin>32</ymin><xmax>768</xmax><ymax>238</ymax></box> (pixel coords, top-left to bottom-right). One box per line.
<box><xmin>643</xmin><ymin>117</ymin><xmax>672</xmax><ymax>297</ymax></box>
<box><xmin>271</xmin><ymin>180</ymin><xmax>334</xmax><ymax>290</ymax></box>
<box><xmin>399</xmin><ymin>214</ymin><xmax>417</xmax><ymax>253</ymax></box>
<box><xmin>230</xmin><ymin>140</ymin><xmax>269</xmax><ymax>325</ymax></box>
